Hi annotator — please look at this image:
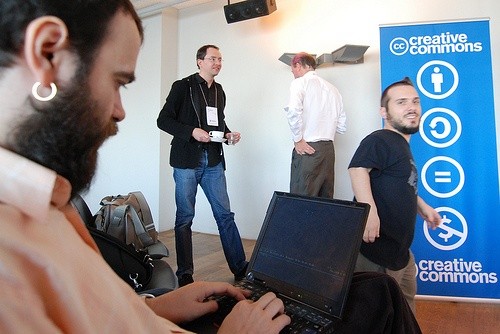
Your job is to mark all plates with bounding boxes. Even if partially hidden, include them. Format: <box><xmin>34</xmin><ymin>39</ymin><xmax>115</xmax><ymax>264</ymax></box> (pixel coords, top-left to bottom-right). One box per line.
<box><xmin>210</xmin><ymin>137</ymin><xmax>228</xmax><ymax>143</ymax></box>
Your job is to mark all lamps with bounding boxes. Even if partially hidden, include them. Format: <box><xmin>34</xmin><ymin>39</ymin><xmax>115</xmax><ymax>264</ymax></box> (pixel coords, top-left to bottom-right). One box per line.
<box><xmin>276</xmin><ymin>43</ymin><xmax>370</xmax><ymax>68</ymax></box>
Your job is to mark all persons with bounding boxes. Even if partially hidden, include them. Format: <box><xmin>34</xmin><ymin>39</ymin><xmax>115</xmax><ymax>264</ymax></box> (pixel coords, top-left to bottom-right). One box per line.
<box><xmin>285</xmin><ymin>52</ymin><xmax>346</xmax><ymax>200</ymax></box>
<box><xmin>348</xmin><ymin>77</ymin><xmax>441</xmax><ymax>316</ymax></box>
<box><xmin>0</xmin><ymin>0</ymin><xmax>422</xmax><ymax>334</ymax></box>
<box><xmin>157</xmin><ymin>45</ymin><xmax>250</xmax><ymax>288</ymax></box>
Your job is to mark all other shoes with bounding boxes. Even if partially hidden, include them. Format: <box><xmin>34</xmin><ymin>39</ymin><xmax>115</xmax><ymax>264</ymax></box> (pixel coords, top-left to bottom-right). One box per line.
<box><xmin>234</xmin><ymin>262</ymin><xmax>250</xmax><ymax>277</ymax></box>
<box><xmin>178</xmin><ymin>274</ymin><xmax>195</xmax><ymax>287</ymax></box>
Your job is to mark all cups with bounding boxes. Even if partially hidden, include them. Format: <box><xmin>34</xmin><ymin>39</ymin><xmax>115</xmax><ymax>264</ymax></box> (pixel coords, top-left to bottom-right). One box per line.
<box><xmin>227</xmin><ymin>134</ymin><xmax>236</xmax><ymax>145</ymax></box>
<box><xmin>208</xmin><ymin>130</ymin><xmax>224</xmax><ymax>139</ymax></box>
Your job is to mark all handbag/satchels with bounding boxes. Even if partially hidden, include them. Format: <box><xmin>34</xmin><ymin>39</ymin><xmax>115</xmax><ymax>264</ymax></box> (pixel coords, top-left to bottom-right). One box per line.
<box><xmin>86</xmin><ymin>225</ymin><xmax>154</xmax><ymax>293</ymax></box>
<box><xmin>95</xmin><ymin>191</ymin><xmax>158</xmax><ymax>247</ymax></box>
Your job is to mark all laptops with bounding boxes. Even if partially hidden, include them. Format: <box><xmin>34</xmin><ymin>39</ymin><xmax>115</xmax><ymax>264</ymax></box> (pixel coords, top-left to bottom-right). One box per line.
<box><xmin>183</xmin><ymin>189</ymin><xmax>372</xmax><ymax>334</ymax></box>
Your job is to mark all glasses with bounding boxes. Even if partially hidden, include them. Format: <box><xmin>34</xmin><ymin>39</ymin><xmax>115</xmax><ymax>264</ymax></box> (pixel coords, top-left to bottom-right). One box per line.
<box><xmin>205</xmin><ymin>57</ymin><xmax>224</xmax><ymax>63</ymax></box>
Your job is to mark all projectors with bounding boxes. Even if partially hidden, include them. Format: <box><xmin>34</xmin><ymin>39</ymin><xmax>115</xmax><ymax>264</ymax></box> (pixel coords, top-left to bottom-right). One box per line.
<box><xmin>223</xmin><ymin>0</ymin><xmax>277</xmax><ymax>24</ymax></box>
